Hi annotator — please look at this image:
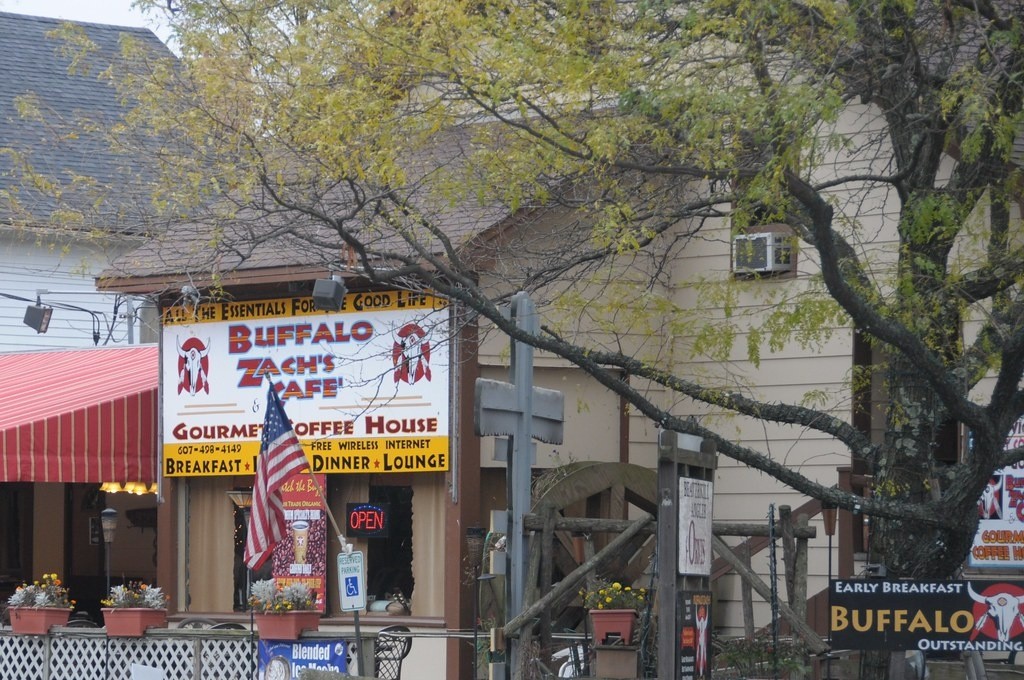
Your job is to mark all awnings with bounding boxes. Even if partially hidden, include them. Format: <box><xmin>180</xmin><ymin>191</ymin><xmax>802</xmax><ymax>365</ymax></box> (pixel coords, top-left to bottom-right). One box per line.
<box><xmin>0</xmin><ymin>347</ymin><xmax>159</xmax><ymax>483</ymax></box>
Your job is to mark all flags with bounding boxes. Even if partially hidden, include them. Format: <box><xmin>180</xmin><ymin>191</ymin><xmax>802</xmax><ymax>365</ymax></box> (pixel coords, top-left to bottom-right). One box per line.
<box><xmin>242</xmin><ymin>382</ymin><xmax>310</xmax><ymax>571</ymax></box>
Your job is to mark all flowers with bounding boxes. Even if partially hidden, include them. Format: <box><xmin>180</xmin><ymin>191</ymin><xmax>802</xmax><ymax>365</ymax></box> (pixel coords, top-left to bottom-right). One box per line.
<box><xmin>129</xmin><ymin>581</ymin><xmax>170</xmax><ymax>609</ymax></box>
<box><xmin>100</xmin><ymin>585</ymin><xmax>148</xmax><ymax>607</ymax></box>
<box><xmin>578</xmin><ymin>574</ymin><xmax>646</xmax><ymax>610</ymax></box>
<box><xmin>7</xmin><ymin>572</ymin><xmax>75</xmax><ymax>612</ymax></box>
<box><xmin>246</xmin><ymin>577</ymin><xmax>320</xmax><ymax>615</ymax></box>
<box><xmin>101</xmin><ymin>507</ymin><xmax>116</xmax><ymax>591</ymax></box>
<box><xmin>590</xmin><ymin>608</ymin><xmax>638</xmax><ymax>646</ymax></box>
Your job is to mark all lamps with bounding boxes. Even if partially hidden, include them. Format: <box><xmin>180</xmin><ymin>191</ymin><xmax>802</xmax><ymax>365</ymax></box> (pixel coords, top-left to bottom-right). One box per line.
<box><xmin>106</xmin><ymin>482</ymin><xmax>123</xmax><ymax>493</ymax></box>
<box><xmin>23</xmin><ymin>289</ymin><xmax>124</xmax><ymax>334</ymax></box>
<box><xmin>147</xmin><ymin>483</ymin><xmax>158</xmax><ymax>494</ymax></box>
<box><xmin>821</xmin><ymin>502</ymin><xmax>838</xmax><ymax>538</ymax></box>
<box><xmin>123</xmin><ymin>481</ymin><xmax>135</xmax><ymax>495</ymax></box>
<box><xmin>312</xmin><ymin>262</ymin><xmax>418</xmax><ymax>314</ymax></box>
<box><xmin>98</xmin><ymin>482</ymin><xmax>111</xmax><ymax>493</ymax></box>
<box><xmin>132</xmin><ymin>482</ymin><xmax>149</xmax><ymax>496</ymax></box>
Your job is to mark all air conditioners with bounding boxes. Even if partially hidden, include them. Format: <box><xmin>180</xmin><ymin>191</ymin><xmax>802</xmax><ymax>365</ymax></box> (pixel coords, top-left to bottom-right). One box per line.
<box><xmin>733</xmin><ymin>233</ymin><xmax>791</xmax><ymax>279</ymax></box>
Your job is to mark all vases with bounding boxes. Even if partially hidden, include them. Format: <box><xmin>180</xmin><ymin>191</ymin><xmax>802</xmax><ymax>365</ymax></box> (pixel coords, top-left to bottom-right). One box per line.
<box><xmin>7</xmin><ymin>607</ymin><xmax>72</xmax><ymax>634</ymax></box>
<box><xmin>255</xmin><ymin>609</ymin><xmax>326</xmax><ymax>639</ymax></box>
<box><xmin>102</xmin><ymin>607</ymin><xmax>150</xmax><ymax>634</ymax></box>
<box><xmin>146</xmin><ymin>608</ymin><xmax>167</xmax><ymax>628</ymax></box>
